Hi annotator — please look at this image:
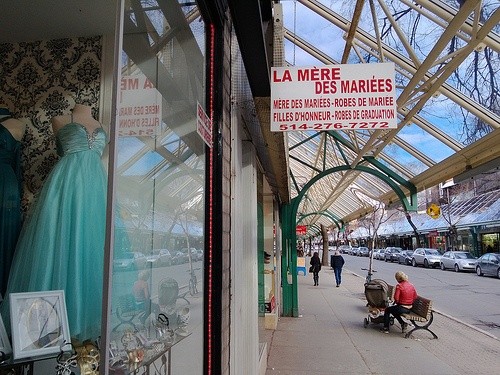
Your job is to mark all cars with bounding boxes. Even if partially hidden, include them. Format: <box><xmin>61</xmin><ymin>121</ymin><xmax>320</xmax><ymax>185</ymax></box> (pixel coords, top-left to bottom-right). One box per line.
<box><xmin>340</xmin><ymin>246</ymin><xmax>402</xmax><ymax>262</ymax></box>
<box><xmin>474</xmin><ymin>252</ymin><xmax>500</xmax><ymax>278</ymax></box>
<box><xmin>397</xmin><ymin>250</ymin><xmax>414</xmax><ymax>264</ymax></box>
<box><xmin>113</xmin><ymin>252</ymin><xmax>146</xmax><ymax>272</ymax></box>
<box><xmin>172</xmin><ymin>248</ymin><xmax>203</xmax><ymax>265</ymax></box>
<box><xmin>411</xmin><ymin>247</ymin><xmax>442</xmax><ymax>268</ymax></box>
<box><xmin>440</xmin><ymin>250</ymin><xmax>479</xmax><ymax>271</ymax></box>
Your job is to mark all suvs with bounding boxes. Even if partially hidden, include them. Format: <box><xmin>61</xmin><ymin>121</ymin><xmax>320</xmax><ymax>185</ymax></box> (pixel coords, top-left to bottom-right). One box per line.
<box><xmin>146</xmin><ymin>249</ymin><xmax>172</xmax><ymax>267</ymax></box>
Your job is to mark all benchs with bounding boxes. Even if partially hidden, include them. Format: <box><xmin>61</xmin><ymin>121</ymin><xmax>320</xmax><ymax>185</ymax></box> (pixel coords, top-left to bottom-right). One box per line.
<box><xmin>401</xmin><ymin>296</ymin><xmax>439</xmax><ymax>340</ymax></box>
<box><xmin>387</xmin><ymin>284</ymin><xmax>396</xmax><ymax>303</ymax></box>
<box><xmin>112</xmin><ymin>293</ymin><xmax>146</xmax><ymax>332</ymax></box>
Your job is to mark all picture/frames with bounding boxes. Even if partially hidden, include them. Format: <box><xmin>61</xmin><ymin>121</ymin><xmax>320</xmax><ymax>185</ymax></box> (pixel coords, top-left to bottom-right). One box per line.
<box><xmin>8</xmin><ymin>290</ymin><xmax>72</xmax><ymax>360</ymax></box>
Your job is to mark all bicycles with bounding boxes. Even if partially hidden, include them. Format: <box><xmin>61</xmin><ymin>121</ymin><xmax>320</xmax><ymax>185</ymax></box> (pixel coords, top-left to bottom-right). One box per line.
<box><xmin>361</xmin><ymin>267</ymin><xmax>377</xmax><ymax>284</ymax></box>
<box><xmin>187</xmin><ymin>268</ymin><xmax>201</xmax><ymax>296</ymax></box>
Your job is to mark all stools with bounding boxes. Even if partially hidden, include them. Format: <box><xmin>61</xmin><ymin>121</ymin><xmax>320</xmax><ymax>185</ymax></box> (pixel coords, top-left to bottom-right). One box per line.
<box><xmin>297</xmin><ymin>267</ymin><xmax>306</xmax><ymax>276</ymax></box>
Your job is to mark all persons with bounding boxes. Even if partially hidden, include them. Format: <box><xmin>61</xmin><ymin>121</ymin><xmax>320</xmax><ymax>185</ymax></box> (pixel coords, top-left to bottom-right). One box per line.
<box><xmin>331</xmin><ymin>251</ymin><xmax>345</xmax><ymax>287</ymax></box>
<box><xmin>310</xmin><ymin>252</ymin><xmax>321</xmax><ymax>286</ymax></box>
<box><xmin>0</xmin><ymin>104</ymin><xmax>26</xmax><ymax>352</ymax></box>
<box><xmin>383</xmin><ymin>272</ymin><xmax>417</xmax><ymax>334</ymax></box>
<box><xmin>4</xmin><ymin>106</ymin><xmax>147</xmax><ymax>344</ymax></box>
<box><xmin>133</xmin><ymin>271</ymin><xmax>160</xmax><ymax>329</ymax></box>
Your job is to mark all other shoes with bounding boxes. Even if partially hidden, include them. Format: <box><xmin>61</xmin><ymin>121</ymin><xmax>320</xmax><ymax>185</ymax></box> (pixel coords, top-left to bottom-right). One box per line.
<box><xmin>401</xmin><ymin>322</ymin><xmax>412</xmax><ymax>333</ymax></box>
<box><xmin>380</xmin><ymin>328</ymin><xmax>389</xmax><ymax>333</ymax></box>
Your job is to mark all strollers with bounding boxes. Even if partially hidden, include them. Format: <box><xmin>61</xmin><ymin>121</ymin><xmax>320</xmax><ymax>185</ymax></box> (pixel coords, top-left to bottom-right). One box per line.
<box><xmin>156</xmin><ymin>278</ymin><xmax>179</xmax><ymax>321</ymax></box>
<box><xmin>363</xmin><ymin>279</ymin><xmax>394</xmax><ymax>328</ymax></box>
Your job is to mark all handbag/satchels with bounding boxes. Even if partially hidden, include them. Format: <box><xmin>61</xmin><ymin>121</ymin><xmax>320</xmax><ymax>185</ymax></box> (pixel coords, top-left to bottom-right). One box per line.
<box><xmin>309</xmin><ymin>266</ymin><xmax>315</xmax><ymax>273</ymax></box>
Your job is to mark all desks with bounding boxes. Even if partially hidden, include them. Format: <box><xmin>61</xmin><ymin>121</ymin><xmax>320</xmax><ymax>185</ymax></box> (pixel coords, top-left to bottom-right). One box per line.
<box><xmin>55</xmin><ymin>330</ymin><xmax>193</xmax><ymax>375</ymax></box>
